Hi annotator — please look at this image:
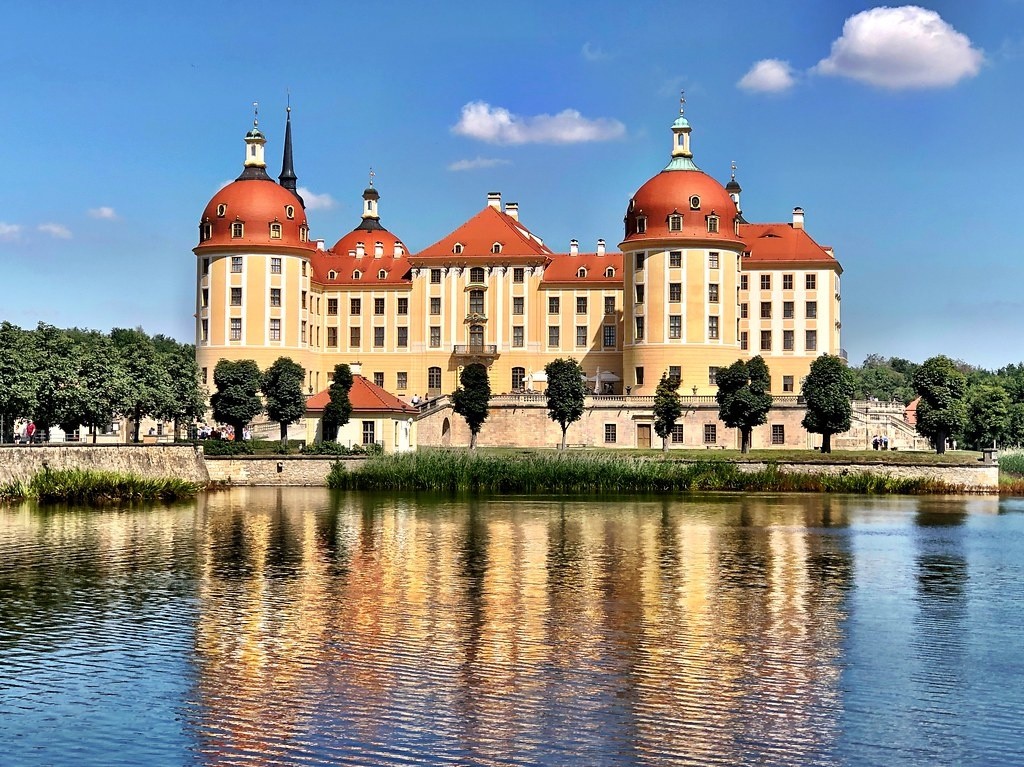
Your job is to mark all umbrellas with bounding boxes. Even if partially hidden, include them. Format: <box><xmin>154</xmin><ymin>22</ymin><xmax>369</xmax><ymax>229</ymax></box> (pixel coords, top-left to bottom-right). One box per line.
<box><xmin>522</xmin><ymin>369</ymin><xmax>622</xmax><ymax>391</ymax></box>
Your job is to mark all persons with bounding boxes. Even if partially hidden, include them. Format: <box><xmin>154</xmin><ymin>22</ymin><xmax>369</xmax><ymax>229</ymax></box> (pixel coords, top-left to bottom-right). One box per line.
<box><xmin>411</xmin><ymin>393</ymin><xmax>429</xmax><ymax>406</ymax></box>
<box><xmin>952</xmin><ymin>438</ymin><xmax>957</xmax><ymax>450</ymax></box>
<box><xmin>605</xmin><ymin>383</ymin><xmax>614</xmax><ymax>394</ymax></box>
<box><xmin>872</xmin><ymin>435</ymin><xmax>899</xmax><ymax>451</ymax></box>
<box><xmin>869</xmin><ymin>395</ymin><xmax>879</xmax><ymax>401</ymax></box>
<box><xmin>129</xmin><ymin>426</ymin><xmax>157</xmax><ymax>443</ymax></box>
<box><xmin>197</xmin><ymin>423</ymin><xmax>252</xmax><ymax>440</ymax></box>
<box><xmin>13</xmin><ymin>419</ymin><xmax>36</xmax><ymax>445</ymax></box>
